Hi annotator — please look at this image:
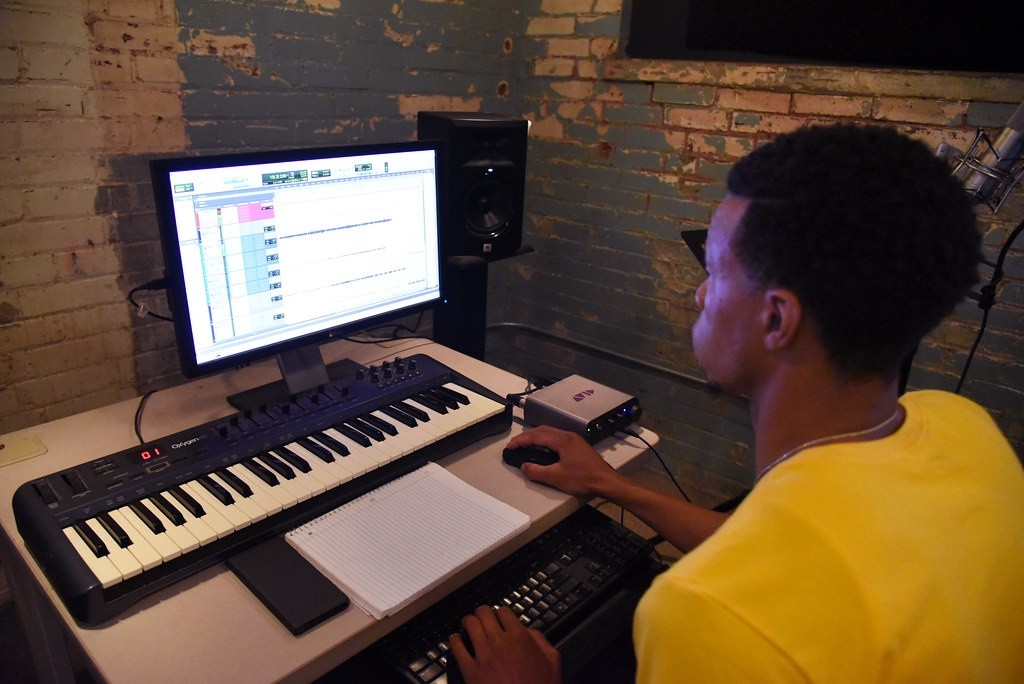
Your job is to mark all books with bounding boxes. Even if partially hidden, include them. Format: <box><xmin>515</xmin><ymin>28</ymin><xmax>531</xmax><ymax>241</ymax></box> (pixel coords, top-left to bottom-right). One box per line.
<box><xmin>224</xmin><ymin>461</ymin><xmax>532</xmax><ymax>636</ymax></box>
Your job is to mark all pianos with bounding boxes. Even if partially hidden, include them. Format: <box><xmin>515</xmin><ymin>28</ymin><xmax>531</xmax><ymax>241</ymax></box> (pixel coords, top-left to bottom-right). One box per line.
<box><xmin>9</xmin><ymin>350</ymin><xmax>513</xmax><ymax>631</ymax></box>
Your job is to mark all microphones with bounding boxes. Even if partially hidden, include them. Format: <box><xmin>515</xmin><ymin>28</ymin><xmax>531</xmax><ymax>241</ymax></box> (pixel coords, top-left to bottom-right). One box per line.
<box><xmin>937</xmin><ymin>95</ymin><xmax>1024</xmax><ymax>204</ymax></box>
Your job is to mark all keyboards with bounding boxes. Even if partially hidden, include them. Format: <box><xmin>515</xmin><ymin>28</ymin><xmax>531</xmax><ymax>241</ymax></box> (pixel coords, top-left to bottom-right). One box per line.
<box><xmin>367</xmin><ymin>505</ymin><xmax>656</xmax><ymax>684</ymax></box>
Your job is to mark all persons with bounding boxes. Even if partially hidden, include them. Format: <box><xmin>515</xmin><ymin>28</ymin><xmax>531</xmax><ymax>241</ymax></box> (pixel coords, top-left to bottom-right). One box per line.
<box><xmin>446</xmin><ymin>118</ymin><xmax>1023</xmax><ymax>684</ymax></box>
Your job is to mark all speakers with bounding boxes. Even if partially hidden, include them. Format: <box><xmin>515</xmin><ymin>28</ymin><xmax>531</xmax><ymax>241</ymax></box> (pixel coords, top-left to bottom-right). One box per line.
<box><xmin>416</xmin><ymin>109</ymin><xmax>528</xmax><ymax>257</ymax></box>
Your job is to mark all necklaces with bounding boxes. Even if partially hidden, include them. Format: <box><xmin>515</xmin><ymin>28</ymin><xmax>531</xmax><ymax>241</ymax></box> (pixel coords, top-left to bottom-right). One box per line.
<box><xmin>755</xmin><ymin>408</ymin><xmax>900</xmax><ymax>484</ymax></box>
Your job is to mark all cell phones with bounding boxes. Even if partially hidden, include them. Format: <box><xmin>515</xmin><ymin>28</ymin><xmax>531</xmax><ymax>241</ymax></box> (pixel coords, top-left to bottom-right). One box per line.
<box><xmin>224</xmin><ymin>536</ymin><xmax>350</xmax><ymax>637</ymax></box>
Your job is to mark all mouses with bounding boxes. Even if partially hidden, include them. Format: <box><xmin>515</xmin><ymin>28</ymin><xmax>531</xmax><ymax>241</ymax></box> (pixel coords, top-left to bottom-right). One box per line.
<box><xmin>503</xmin><ymin>442</ymin><xmax>561</xmax><ymax>470</ymax></box>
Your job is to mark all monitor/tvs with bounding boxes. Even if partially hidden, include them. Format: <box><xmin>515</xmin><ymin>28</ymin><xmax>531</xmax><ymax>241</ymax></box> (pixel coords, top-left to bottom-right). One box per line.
<box><xmin>147</xmin><ymin>139</ymin><xmax>449</xmax><ymax>412</ymax></box>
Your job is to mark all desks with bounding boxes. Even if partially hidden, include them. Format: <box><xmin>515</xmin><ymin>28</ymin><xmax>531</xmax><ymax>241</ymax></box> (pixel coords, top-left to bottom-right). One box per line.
<box><xmin>0</xmin><ymin>335</ymin><xmax>659</xmax><ymax>684</ymax></box>
